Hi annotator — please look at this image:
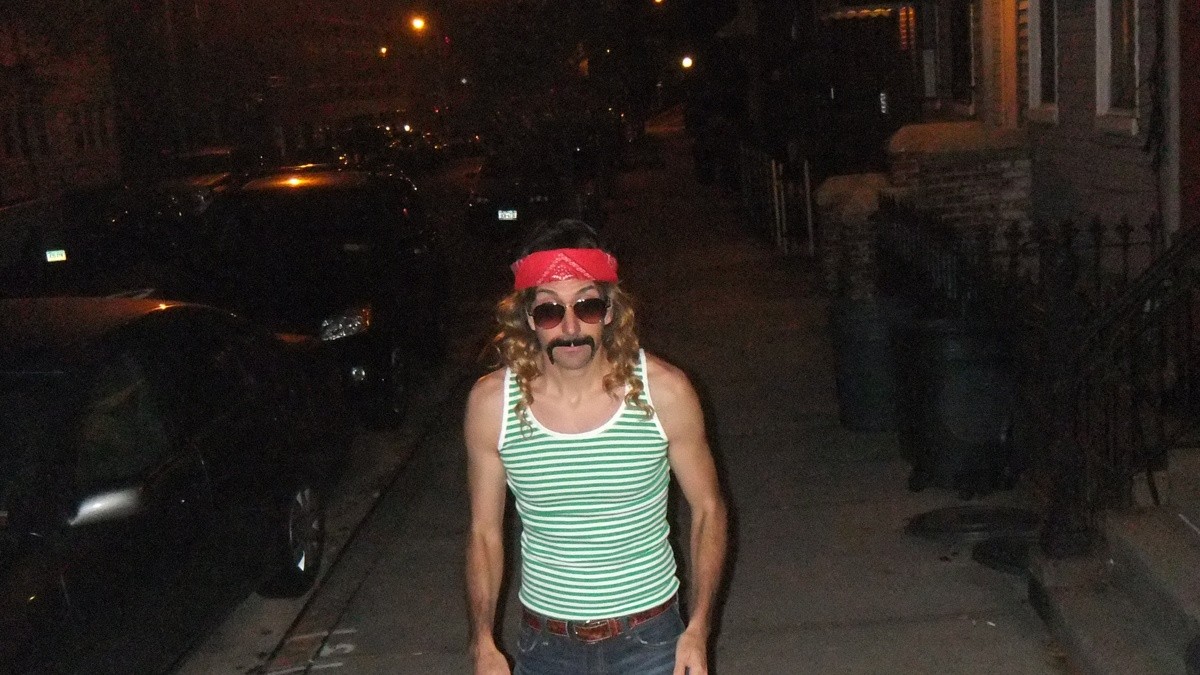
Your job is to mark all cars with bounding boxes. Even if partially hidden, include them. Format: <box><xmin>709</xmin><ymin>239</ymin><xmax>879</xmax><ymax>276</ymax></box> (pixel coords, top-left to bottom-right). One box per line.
<box><xmin>0</xmin><ymin>296</ymin><xmax>357</xmax><ymax>674</ymax></box>
<box><xmin>98</xmin><ymin>97</ymin><xmax>644</xmax><ymax>430</ymax></box>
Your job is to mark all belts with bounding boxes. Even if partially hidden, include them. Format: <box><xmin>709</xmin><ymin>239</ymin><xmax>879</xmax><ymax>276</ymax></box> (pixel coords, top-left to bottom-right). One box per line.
<box><xmin>522</xmin><ymin>594</ymin><xmax>677</xmax><ymax>644</ymax></box>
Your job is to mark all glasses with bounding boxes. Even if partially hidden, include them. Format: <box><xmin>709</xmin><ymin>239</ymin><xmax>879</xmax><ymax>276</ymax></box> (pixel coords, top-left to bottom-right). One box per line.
<box><xmin>527</xmin><ymin>297</ymin><xmax>611</xmax><ymax>330</ymax></box>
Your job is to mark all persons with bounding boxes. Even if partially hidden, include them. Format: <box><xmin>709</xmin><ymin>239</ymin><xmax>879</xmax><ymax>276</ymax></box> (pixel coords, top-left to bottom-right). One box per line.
<box><xmin>462</xmin><ymin>217</ymin><xmax>730</xmax><ymax>675</ymax></box>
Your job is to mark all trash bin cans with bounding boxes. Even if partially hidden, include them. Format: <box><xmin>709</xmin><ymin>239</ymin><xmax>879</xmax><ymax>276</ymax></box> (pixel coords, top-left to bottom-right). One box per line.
<box><xmin>889</xmin><ymin>307</ymin><xmax>1018</xmax><ymax>495</ymax></box>
<box><xmin>832</xmin><ymin>300</ymin><xmax>896</xmax><ymax>433</ymax></box>
